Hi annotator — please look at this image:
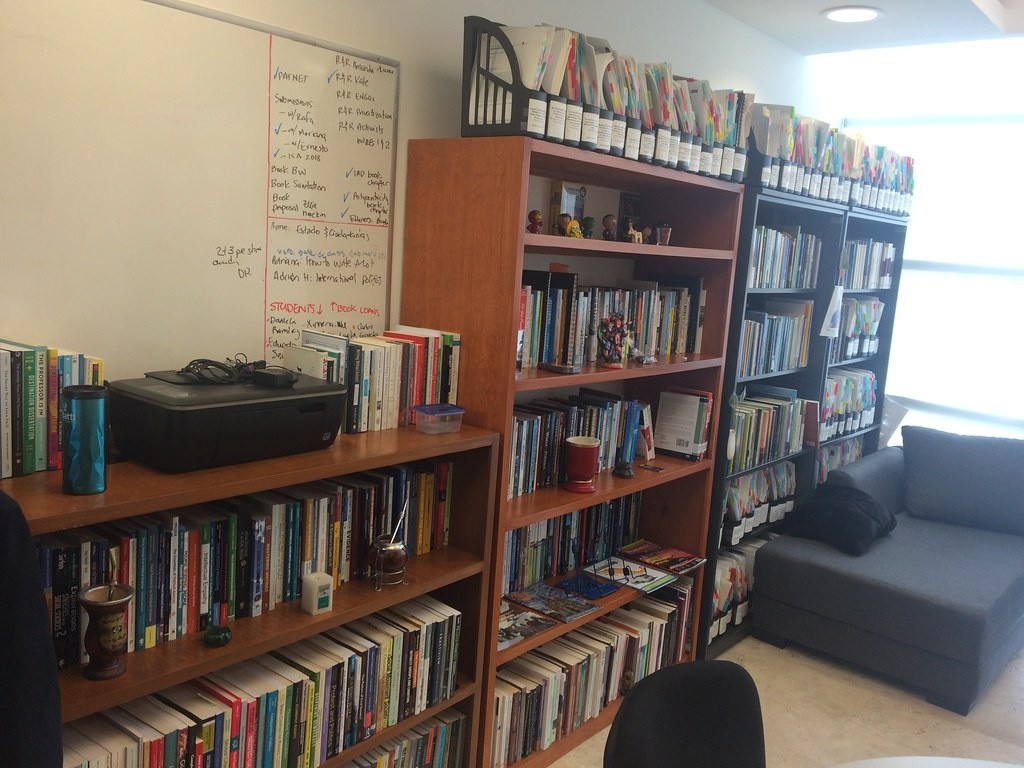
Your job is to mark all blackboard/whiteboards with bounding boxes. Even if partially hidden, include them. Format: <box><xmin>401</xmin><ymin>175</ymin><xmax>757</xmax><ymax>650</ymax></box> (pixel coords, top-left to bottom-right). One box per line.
<box><xmin>0</xmin><ymin>0</ymin><xmax>402</xmax><ymax>386</ymax></box>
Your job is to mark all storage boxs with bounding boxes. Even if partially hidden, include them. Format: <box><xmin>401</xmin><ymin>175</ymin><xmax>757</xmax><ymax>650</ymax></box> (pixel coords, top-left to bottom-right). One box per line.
<box><xmin>548</xmin><ymin>178</ymin><xmax>586</xmax><ymax>238</ymax></box>
<box><xmin>415</xmin><ymin>403</ymin><xmax>465</xmax><ymax>435</ymax></box>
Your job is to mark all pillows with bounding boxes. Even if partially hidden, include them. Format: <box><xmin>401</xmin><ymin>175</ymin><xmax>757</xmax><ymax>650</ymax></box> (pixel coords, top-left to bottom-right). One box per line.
<box><xmin>784</xmin><ymin>482</ymin><xmax>898</xmax><ymax>555</ymax></box>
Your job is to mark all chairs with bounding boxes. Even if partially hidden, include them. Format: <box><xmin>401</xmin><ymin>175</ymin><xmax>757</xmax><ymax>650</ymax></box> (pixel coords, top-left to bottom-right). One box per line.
<box><xmin>603</xmin><ymin>659</ymin><xmax>765</xmax><ymax>768</ymax></box>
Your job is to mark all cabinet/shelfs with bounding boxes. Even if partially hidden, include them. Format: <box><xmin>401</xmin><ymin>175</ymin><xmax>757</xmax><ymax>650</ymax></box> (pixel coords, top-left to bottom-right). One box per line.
<box><xmin>0</xmin><ymin>427</ymin><xmax>501</xmax><ymax>768</ymax></box>
<box><xmin>400</xmin><ymin>137</ymin><xmax>746</xmax><ymax>768</ymax></box>
<box><xmin>695</xmin><ymin>191</ymin><xmax>908</xmax><ymax>660</ymax></box>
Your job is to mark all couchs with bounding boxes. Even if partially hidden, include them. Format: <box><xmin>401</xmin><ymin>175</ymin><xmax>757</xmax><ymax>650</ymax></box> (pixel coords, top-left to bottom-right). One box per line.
<box><xmin>746</xmin><ymin>426</ymin><xmax>1024</xmax><ymax>716</ymax></box>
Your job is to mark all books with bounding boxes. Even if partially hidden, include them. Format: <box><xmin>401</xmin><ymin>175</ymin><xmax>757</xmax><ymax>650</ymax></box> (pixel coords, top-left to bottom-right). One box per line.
<box><xmin>0</xmin><ymin>325</ymin><xmax>470</xmax><ymax>768</ymax></box>
<box><xmin>468</xmin><ymin>23</ymin><xmax>915</xmax><ymax>768</ymax></box>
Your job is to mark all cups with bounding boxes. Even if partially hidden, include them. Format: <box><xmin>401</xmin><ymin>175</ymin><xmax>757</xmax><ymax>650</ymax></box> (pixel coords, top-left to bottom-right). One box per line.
<box><xmin>62</xmin><ymin>384</ymin><xmax>107</xmax><ymax>496</ymax></box>
<box><xmin>565</xmin><ymin>436</ymin><xmax>600</xmax><ymax>493</ymax></box>
<box><xmin>367</xmin><ymin>535</ymin><xmax>408</xmax><ymax>572</ymax></box>
<box><xmin>657</xmin><ymin>227</ymin><xmax>672</xmax><ymax>245</ymax></box>
